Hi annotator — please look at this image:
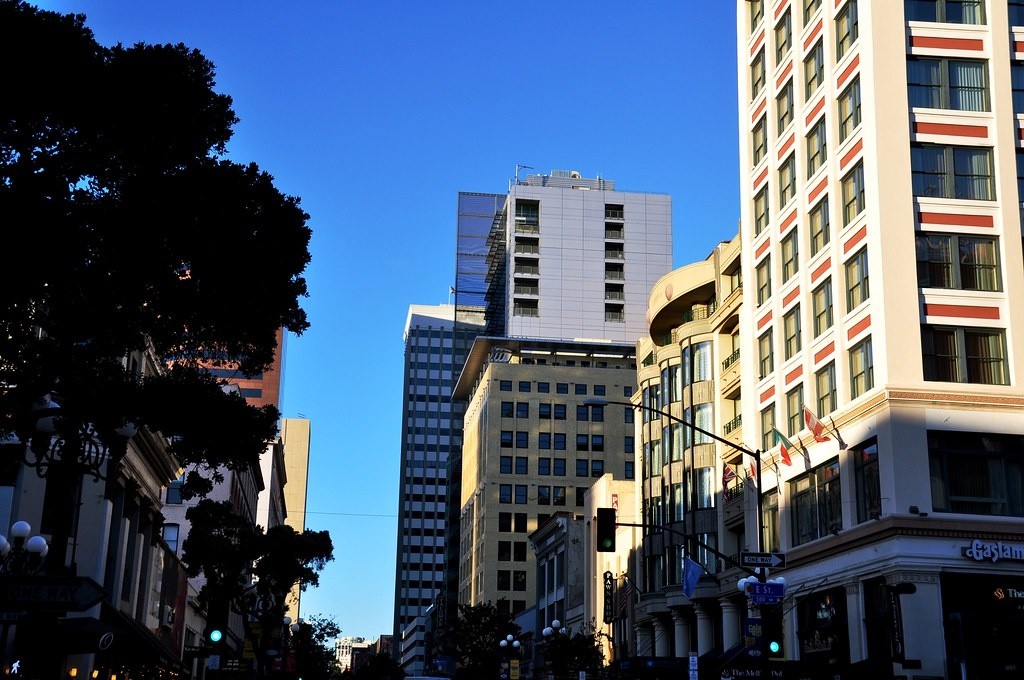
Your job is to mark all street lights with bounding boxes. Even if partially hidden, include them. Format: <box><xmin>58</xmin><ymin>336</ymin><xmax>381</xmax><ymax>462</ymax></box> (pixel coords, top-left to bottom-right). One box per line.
<box><xmin>0</xmin><ymin>519</ymin><xmax>48</xmax><ymax>680</ymax></box>
<box><xmin>541</xmin><ymin>620</ymin><xmax>567</xmax><ymax>680</ymax></box>
<box><xmin>580</xmin><ymin>394</ymin><xmax>771</xmax><ymax>680</ymax></box>
<box><xmin>500</xmin><ymin>634</ymin><xmax>520</xmax><ymax>680</ymax></box>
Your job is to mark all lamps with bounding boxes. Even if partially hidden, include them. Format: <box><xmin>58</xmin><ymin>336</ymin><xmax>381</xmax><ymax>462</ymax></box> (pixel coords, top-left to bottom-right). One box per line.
<box><xmin>871</xmin><ymin>512</ymin><xmax>880</xmax><ymax>521</ymax></box>
<box><xmin>909</xmin><ymin>505</ymin><xmax>920</xmax><ymax>514</ymax></box>
<box><xmin>830</xmin><ymin>523</ymin><xmax>842</xmax><ymax>536</ymax></box>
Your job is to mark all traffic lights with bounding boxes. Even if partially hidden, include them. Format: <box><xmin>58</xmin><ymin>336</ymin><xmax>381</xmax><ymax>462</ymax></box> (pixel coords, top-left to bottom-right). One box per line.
<box><xmin>761</xmin><ymin>603</ymin><xmax>785</xmax><ymax>659</ymax></box>
<box><xmin>203</xmin><ymin>598</ymin><xmax>230</xmax><ymax>649</ymax></box>
<box><xmin>7</xmin><ymin>653</ymin><xmax>28</xmax><ymax>678</ymax></box>
<box><xmin>596</xmin><ymin>509</ymin><xmax>616</xmax><ymax>552</ymax></box>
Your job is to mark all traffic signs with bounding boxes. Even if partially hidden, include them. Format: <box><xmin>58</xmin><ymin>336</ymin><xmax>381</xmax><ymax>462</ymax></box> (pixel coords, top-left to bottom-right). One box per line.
<box><xmin>740</xmin><ymin>551</ymin><xmax>787</xmax><ymax>569</ymax></box>
